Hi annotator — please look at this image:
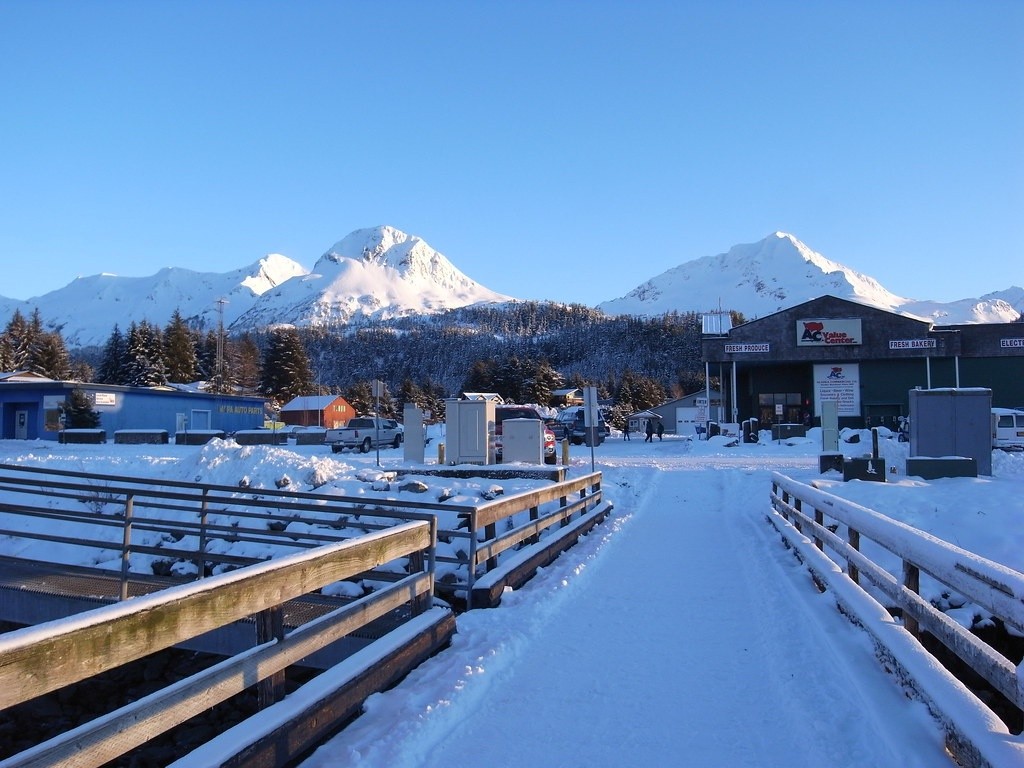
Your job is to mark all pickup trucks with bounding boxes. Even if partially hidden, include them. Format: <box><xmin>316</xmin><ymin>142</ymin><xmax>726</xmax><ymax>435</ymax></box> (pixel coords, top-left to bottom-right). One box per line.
<box><xmin>323</xmin><ymin>417</ymin><xmax>401</xmax><ymax>453</ymax></box>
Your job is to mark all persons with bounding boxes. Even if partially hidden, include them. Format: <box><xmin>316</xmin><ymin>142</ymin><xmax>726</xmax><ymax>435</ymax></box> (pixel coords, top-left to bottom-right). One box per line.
<box><xmin>657</xmin><ymin>422</ymin><xmax>664</xmax><ymax>441</ymax></box>
<box><xmin>545</xmin><ymin>418</ymin><xmax>554</xmax><ymax>431</ymax></box>
<box><xmin>624</xmin><ymin>422</ymin><xmax>630</xmax><ymax>441</ymax></box>
<box><xmin>645</xmin><ymin>419</ymin><xmax>653</xmax><ymax>443</ymax></box>
<box><xmin>802</xmin><ymin>410</ymin><xmax>811</xmax><ymax>427</ymax></box>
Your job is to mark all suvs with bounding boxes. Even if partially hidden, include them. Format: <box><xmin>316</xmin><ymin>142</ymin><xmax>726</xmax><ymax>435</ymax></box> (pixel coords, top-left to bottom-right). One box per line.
<box><xmin>495</xmin><ymin>405</ymin><xmax>556</xmax><ymax>465</ymax></box>
<box><xmin>554</xmin><ymin>406</ymin><xmax>606</xmax><ymax>444</ymax></box>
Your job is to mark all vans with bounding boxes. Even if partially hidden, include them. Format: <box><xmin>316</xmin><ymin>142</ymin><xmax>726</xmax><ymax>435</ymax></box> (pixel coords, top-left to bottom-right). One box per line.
<box><xmin>991</xmin><ymin>407</ymin><xmax>1024</xmax><ymax>450</ymax></box>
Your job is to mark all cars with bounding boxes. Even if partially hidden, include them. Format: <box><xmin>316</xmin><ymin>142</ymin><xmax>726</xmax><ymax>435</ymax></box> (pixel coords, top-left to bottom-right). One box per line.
<box><xmin>897</xmin><ymin>411</ymin><xmax>910</xmax><ymax>443</ymax></box>
<box><xmin>388</xmin><ymin>420</ymin><xmax>405</xmax><ymax>443</ymax></box>
